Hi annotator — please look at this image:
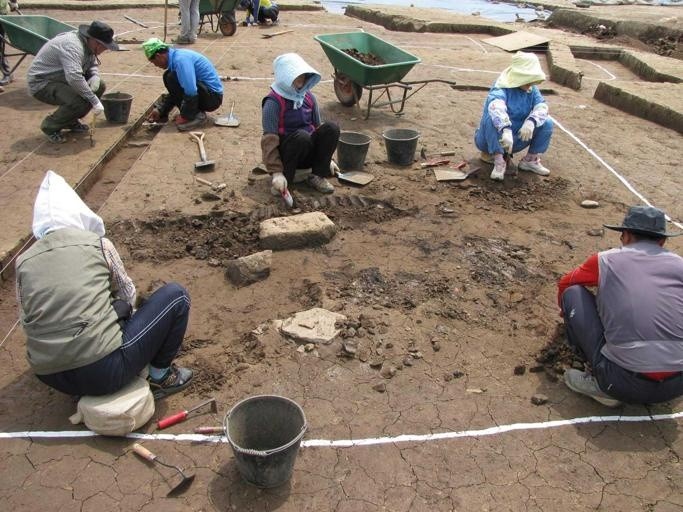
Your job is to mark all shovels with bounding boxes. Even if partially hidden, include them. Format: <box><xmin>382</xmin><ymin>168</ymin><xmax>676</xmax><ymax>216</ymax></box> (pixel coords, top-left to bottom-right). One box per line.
<box><xmin>282</xmin><ymin>189</ymin><xmax>293</xmax><ymax>207</ymax></box>
<box><xmin>163</xmin><ymin>0</ymin><xmax>168</xmax><ymax>42</ymax></box>
<box><xmin>190</xmin><ymin>131</ymin><xmax>215</xmax><ymax>170</ymax></box>
<box><xmin>433</xmin><ymin>162</ymin><xmax>481</xmax><ymax>181</ymax></box>
<box><xmin>334</xmin><ymin>171</ymin><xmax>374</xmax><ymax>186</ymax></box>
<box><xmin>216</xmin><ymin>100</ymin><xmax>241</xmax><ymax>127</ymax></box>
<box><xmin>261</xmin><ymin>30</ymin><xmax>295</xmax><ymax>37</ymax></box>
<box><xmin>421</xmin><ymin>159</ymin><xmax>450</xmax><ymax>166</ymax></box>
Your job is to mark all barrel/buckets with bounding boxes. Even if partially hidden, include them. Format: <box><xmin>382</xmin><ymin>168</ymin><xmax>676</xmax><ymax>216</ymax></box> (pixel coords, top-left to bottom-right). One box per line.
<box><xmin>335</xmin><ymin>129</ymin><xmax>373</xmax><ymax>170</ymax></box>
<box><xmin>102</xmin><ymin>91</ymin><xmax>134</xmax><ymax>125</ymax></box>
<box><xmin>382</xmin><ymin>126</ymin><xmax>421</xmax><ymax>167</ymax></box>
<box><xmin>221</xmin><ymin>392</ymin><xmax>310</xmax><ymax>491</ymax></box>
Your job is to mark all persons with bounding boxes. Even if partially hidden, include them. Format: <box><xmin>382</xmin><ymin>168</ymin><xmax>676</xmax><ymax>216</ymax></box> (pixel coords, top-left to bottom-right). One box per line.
<box><xmin>553</xmin><ymin>204</ymin><xmax>683</xmax><ymax>412</ymax></box>
<box><xmin>12</xmin><ymin>169</ymin><xmax>194</xmax><ymax>401</ymax></box>
<box><xmin>474</xmin><ymin>49</ymin><xmax>554</xmax><ymax>180</ymax></box>
<box><xmin>170</xmin><ymin>0</ymin><xmax>200</xmax><ymax>44</ymax></box>
<box><xmin>25</xmin><ymin>20</ymin><xmax>121</xmax><ymax>144</ymax></box>
<box><xmin>260</xmin><ymin>53</ymin><xmax>340</xmax><ymax>196</ymax></box>
<box><xmin>240</xmin><ymin>0</ymin><xmax>280</xmax><ymax>27</ymax></box>
<box><xmin>0</xmin><ymin>0</ymin><xmax>21</xmax><ymax>87</ymax></box>
<box><xmin>141</xmin><ymin>36</ymin><xmax>224</xmax><ymax>132</ymax></box>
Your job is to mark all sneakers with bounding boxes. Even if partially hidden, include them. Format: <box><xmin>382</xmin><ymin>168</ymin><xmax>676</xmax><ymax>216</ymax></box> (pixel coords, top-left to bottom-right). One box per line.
<box><xmin>188</xmin><ymin>38</ymin><xmax>198</xmax><ymax>44</ymax></box>
<box><xmin>64</xmin><ymin>118</ymin><xmax>89</xmax><ymax>132</ymax></box>
<box><xmin>144</xmin><ymin>364</ymin><xmax>192</xmax><ymax>400</ymax></box>
<box><xmin>271</xmin><ymin>17</ymin><xmax>280</xmax><ymax>26</ymax></box>
<box><xmin>306</xmin><ymin>174</ymin><xmax>334</xmax><ymax>194</ymax></box>
<box><xmin>517</xmin><ymin>156</ymin><xmax>551</xmax><ymax>176</ymax></box>
<box><xmin>40</xmin><ymin>129</ymin><xmax>67</xmax><ymax>144</ymax></box>
<box><xmin>490</xmin><ymin>158</ymin><xmax>507</xmax><ymax>181</ymax></box>
<box><xmin>563</xmin><ymin>367</ymin><xmax>624</xmax><ymax>408</ymax></box>
<box><xmin>177</xmin><ymin>115</ymin><xmax>207</xmax><ymax>131</ymax></box>
<box><xmin>268</xmin><ymin>179</ymin><xmax>294</xmax><ymax>196</ymax></box>
<box><xmin>170</xmin><ymin>34</ymin><xmax>189</xmax><ymax>44</ymax></box>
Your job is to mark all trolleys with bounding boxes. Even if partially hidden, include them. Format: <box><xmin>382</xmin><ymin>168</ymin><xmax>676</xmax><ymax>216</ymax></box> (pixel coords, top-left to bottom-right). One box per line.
<box><xmin>193</xmin><ymin>1</ymin><xmax>240</xmax><ymax>37</ymax></box>
<box><xmin>314</xmin><ymin>31</ymin><xmax>456</xmax><ymax>121</ymax></box>
<box><xmin>0</xmin><ymin>13</ymin><xmax>88</xmax><ymax>83</ymax></box>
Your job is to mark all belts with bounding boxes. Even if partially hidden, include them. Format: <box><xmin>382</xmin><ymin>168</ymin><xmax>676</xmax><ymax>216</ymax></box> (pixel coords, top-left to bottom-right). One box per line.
<box><xmin>626</xmin><ymin>370</ymin><xmax>683</xmax><ymax>384</ymax></box>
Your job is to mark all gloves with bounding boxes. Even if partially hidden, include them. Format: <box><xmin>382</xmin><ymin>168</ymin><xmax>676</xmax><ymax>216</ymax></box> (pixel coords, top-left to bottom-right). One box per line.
<box><xmin>87</xmin><ymin>74</ymin><xmax>100</xmax><ymax>92</ymax></box>
<box><xmin>92</xmin><ymin>101</ymin><xmax>105</xmax><ymax>116</ymax></box>
<box><xmin>247</xmin><ymin>23</ymin><xmax>252</xmax><ymax>27</ymax></box>
<box><xmin>498</xmin><ymin>129</ymin><xmax>514</xmax><ymax>156</ymax></box>
<box><xmin>253</xmin><ymin>22</ymin><xmax>258</xmax><ymax>26</ymax></box>
<box><xmin>175</xmin><ymin>115</ymin><xmax>187</xmax><ymax>124</ymax></box>
<box><xmin>517</xmin><ymin>119</ymin><xmax>535</xmax><ymax>142</ymax></box>
<box><xmin>270</xmin><ymin>171</ymin><xmax>289</xmax><ymax>196</ymax></box>
<box><xmin>146</xmin><ymin>109</ymin><xmax>161</xmax><ymax>123</ymax></box>
<box><xmin>329</xmin><ymin>159</ymin><xmax>340</xmax><ymax>176</ymax></box>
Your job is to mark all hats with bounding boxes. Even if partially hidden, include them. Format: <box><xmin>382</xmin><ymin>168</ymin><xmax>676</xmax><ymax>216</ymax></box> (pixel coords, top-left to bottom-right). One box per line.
<box><xmin>601</xmin><ymin>205</ymin><xmax>683</xmax><ymax>239</ymax></box>
<box><xmin>494</xmin><ymin>52</ymin><xmax>548</xmax><ymax>91</ymax></box>
<box><xmin>77</xmin><ymin>19</ymin><xmax>119</xmax><ymax>51</ymax></box>
<box><xmin>270</xmin><ymin>52</ymin><xmax>323</xmax><ymax>104</ymax></box>
